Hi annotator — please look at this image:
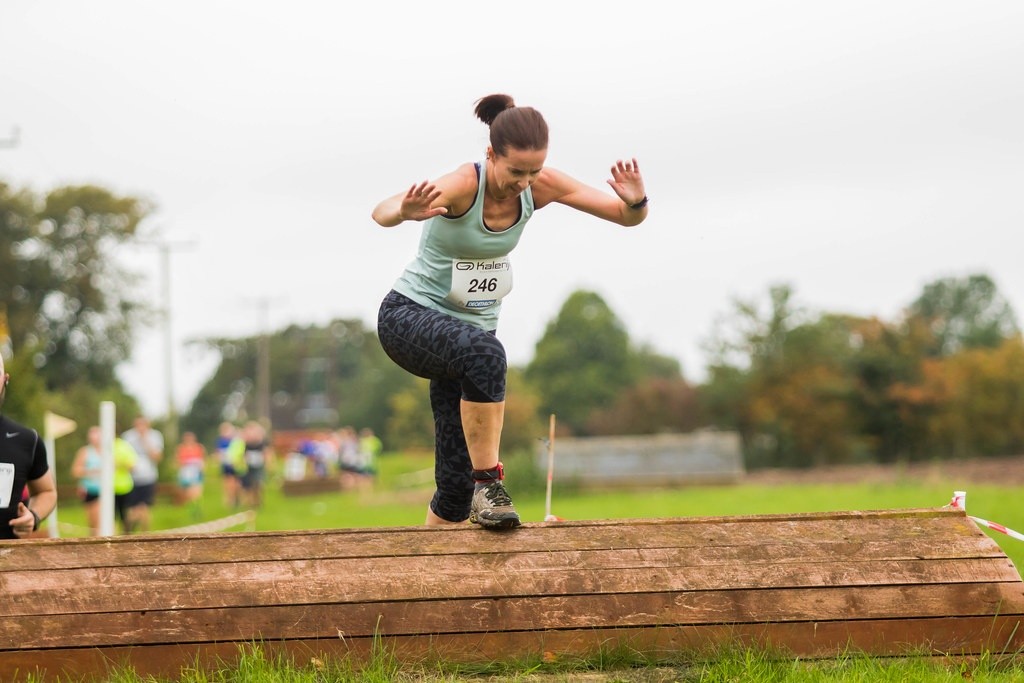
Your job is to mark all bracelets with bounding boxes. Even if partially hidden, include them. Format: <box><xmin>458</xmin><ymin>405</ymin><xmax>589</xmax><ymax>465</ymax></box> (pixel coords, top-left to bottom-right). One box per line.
<box><xmin>30</xmin><ymin>510</ymin><xmax>40</xmax><ymax>531</ymax></box>
<box><xmin>626</xmin><ymin>197</ymin><xmax>648</xmax><ymax>210</ymax></box>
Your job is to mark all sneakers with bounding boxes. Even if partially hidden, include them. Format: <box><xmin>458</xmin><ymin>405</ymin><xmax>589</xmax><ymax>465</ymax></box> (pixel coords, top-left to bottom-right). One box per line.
<box><xmin>469</xmin><ymin>470</ymin><xmax>521</xmax><ymax>527</ymax></box>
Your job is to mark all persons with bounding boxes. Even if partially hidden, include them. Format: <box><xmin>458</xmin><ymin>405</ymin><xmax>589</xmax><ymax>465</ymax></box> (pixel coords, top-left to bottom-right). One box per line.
<box><xmin>0</xmin><ymin>352</ymin><xmax>58</xmax><ymax>540</ymax></box>
<box><xmin>72</xmin><ymin>415</ymin><xmax>383</xmax><ymax>536</ymax></box>
<box><xmin>371</xmin><ymin>95</ymin><xmax>648</xmax><ymax>530</ymax></box>
